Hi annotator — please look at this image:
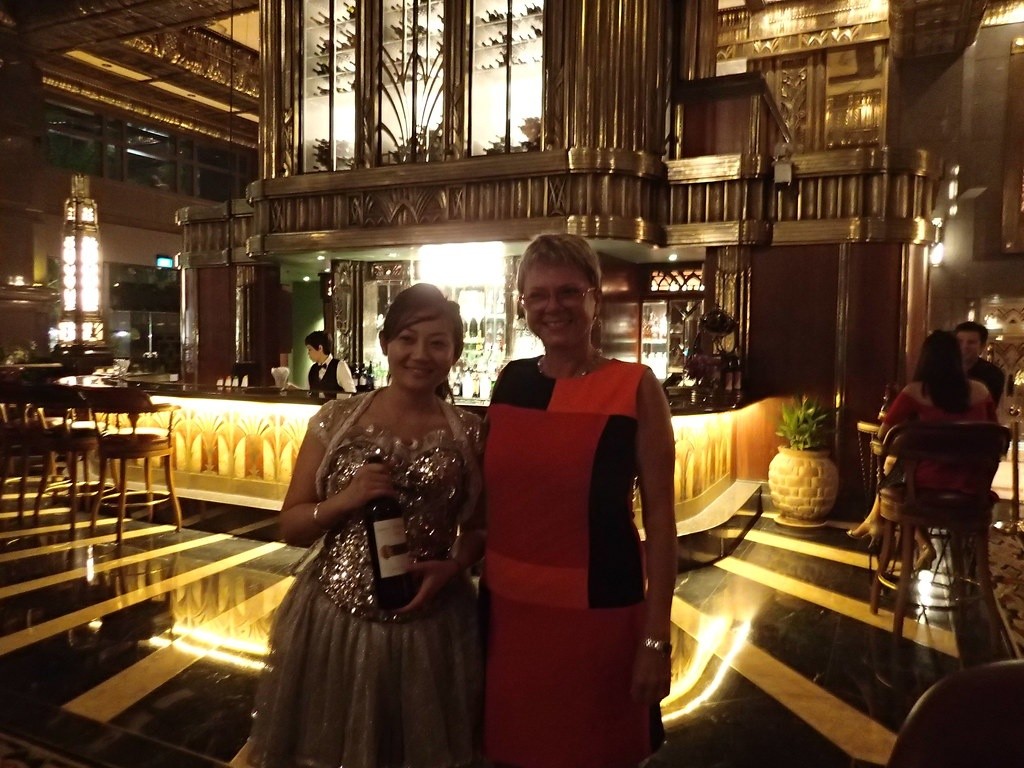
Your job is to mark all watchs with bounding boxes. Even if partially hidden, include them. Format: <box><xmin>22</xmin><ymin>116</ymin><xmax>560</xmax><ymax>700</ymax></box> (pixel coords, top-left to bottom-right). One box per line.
<box><xmin>640</xmin><ymin>635</ymin><xmax>672</xmax><ymax>658</ymax></box>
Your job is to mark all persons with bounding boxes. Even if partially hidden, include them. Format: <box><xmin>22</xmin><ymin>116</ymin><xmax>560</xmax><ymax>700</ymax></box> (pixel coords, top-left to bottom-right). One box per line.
<box><xmin>281</xmin><ymin>332</ymin><xmax>357</xmax><ymax>401</ymax></box>
<box><xmin>244</xmin><ymin>283</ymin><xmax>482</xmax><ymax>768</ymax></box>
<box><xmin>148</xmin><ymin>161</ymin><xmax>172</xmax><ymax>192</ymax></box>
<box><xmin>480</xmin><ymin>229</ymin><xmax>678</xmax><ymax>768</ymax></box>
<box><xmin>846</xmin><ymin>322</ymin><xmax>1006</xmax><ymax>572</ymax></box>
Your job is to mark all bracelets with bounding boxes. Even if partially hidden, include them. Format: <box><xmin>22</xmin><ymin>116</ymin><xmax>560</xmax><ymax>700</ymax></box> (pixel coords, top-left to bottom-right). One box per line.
<box><xmin>313</xmin><ymin>502</ymin><xmax>332</xmax><ymax>534</ymax></box>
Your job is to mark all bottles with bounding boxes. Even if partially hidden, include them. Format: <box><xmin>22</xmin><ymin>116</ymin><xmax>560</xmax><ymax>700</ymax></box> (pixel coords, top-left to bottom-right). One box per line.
<box><xmin>364</xmin><ymin>454</ymin><xmax>415</xmax><ymax>609</ymax></box>
<box><xmin>471</xmin><ymin>375</ymin><xmax>481</xmax><ymax>398</ymax></box>
<box><xmin>724</xmin><ymin>348</ymin><xmax>741</xmax><ymax>390</ymax></box>
<box><xmin>351</xmin><ymin>360</ymin><xmax>374</xmax><ymax>392</ymax></box>
<box><xmin>453</xmin><ymin>374</ymin><xmax>462</xmax><ymax>397</ymax></box>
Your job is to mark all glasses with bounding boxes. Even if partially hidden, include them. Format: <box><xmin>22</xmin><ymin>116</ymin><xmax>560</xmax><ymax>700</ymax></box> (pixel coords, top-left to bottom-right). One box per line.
<box><xmin>519</xmin><ymin>287</ymin><xmax>596</xmax><ymax>311</ymax></box>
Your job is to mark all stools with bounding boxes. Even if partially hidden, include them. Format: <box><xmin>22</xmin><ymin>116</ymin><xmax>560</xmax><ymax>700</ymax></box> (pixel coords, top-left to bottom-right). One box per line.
<box><xmin>0</xmin><ymin>366</ymin><xmax>181</xmax><ymax>544</ymax></box>
<box><xmin>873</xmin><ymin>421</ymin><xmax>1012</xmax><ymax>657</ymax></box>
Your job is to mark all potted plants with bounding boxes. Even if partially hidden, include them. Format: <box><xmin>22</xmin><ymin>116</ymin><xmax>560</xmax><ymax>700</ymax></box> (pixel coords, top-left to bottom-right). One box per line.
<box><xmin>768</xmin><ymin>394</ymin><xmax>845</xmax><ymax>527</ymax></box>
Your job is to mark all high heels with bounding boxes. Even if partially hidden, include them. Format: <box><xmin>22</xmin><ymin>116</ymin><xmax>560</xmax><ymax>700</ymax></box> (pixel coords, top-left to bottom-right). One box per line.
<box><xmin>914</xmin><ymin>547</ymin><xmax>937</xmax><ymax>573</ymax></box>
<box><xmin>846</xmin><ymin>526</ymin><xmax>882</xmax><ymax>549</ymax></box>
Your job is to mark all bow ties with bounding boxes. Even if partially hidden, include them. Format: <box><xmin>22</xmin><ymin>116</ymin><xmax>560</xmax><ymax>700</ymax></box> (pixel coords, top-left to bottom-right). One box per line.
<box><xmin>318</xmin><ymin>363</ymin><xmax>327</xmax><ymax>370</ymax></box>
